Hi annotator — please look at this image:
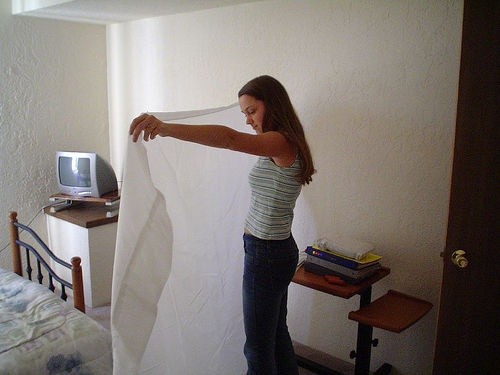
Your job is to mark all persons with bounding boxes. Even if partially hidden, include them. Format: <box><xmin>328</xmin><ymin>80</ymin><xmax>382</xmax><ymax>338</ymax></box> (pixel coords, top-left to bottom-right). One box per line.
<box><xmin>129</xmin><ymin>75</ymin><xmax>315</xmax><ymax>375</ymax></box>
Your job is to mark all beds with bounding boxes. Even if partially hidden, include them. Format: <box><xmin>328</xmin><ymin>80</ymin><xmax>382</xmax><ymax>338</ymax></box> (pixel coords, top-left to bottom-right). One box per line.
<box><xmin>0</xmin><ymin>211</ymin><xmax>111</xmax><ymax>375</ymax></box>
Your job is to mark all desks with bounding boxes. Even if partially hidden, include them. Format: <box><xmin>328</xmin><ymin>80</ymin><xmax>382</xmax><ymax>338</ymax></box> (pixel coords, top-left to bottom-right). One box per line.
<box><xmin>293</xmin><ymin>263</ymin><xmax>434</xmax><ymax>375</ymax></box>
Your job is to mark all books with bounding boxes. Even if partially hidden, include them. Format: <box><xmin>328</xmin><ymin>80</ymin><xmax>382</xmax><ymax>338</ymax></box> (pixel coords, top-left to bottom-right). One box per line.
<box><xmin>304</xmin><ymin>244</ymin><xmax>382</xmax><ymax>284</ymax></box>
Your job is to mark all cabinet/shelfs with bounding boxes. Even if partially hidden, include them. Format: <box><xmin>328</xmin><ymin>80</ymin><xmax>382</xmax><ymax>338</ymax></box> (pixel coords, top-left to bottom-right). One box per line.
<box><xmin>44</xmin><ymin>205</ymin><xmax>119</xmax><ymax>309</ymax></box>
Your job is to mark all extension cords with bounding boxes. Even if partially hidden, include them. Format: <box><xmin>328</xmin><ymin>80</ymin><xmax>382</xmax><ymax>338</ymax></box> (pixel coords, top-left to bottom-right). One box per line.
<box><xmin>51</xmin><ymin>203</ymin><xmax>68</xmax><ymax>212</ymax></box>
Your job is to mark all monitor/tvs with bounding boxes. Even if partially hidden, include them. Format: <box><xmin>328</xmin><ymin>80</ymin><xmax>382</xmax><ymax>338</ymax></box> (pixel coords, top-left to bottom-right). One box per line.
<box><xmin>55</xmin><ymin>150</ymin><xmax>117</xmax><ymax>198</ymax></box>
